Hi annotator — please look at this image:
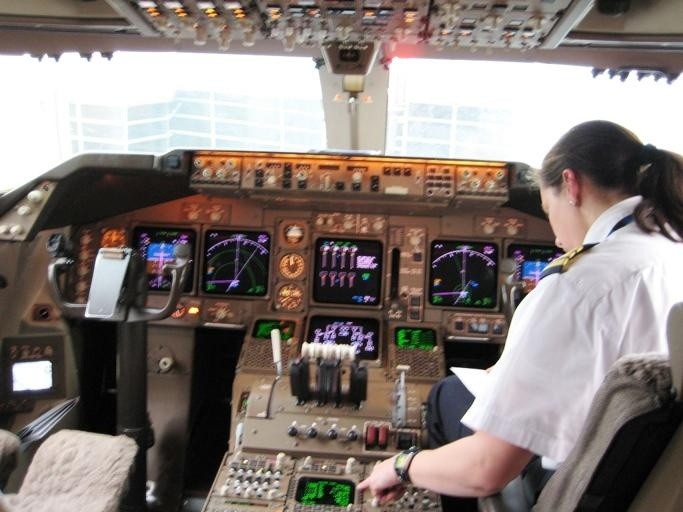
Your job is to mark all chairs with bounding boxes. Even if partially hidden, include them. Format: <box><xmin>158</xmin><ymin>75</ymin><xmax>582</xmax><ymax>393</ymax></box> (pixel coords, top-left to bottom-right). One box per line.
<box><xmin>1</xmin><ymin>430</ymin><xmax>141</xmax><ymax>512</ymax></box>
<box><xmin>475</xmin><ymin>301</ymin><xmax>683</xmax><ymax>510</ymax></box>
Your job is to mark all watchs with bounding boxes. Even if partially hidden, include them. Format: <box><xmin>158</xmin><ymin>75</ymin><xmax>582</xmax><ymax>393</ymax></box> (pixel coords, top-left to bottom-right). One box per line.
<box><xmin>393</xmin><ymin>444</ymin><xmax>424</xmax><ymax>487</ymax></box>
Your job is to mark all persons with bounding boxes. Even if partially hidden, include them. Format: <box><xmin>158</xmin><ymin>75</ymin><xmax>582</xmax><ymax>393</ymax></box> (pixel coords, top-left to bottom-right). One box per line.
<box><xmin>355</xmin><ymin>119</ymin><xmax>683</xmax><ymax>512</ymax></box>
